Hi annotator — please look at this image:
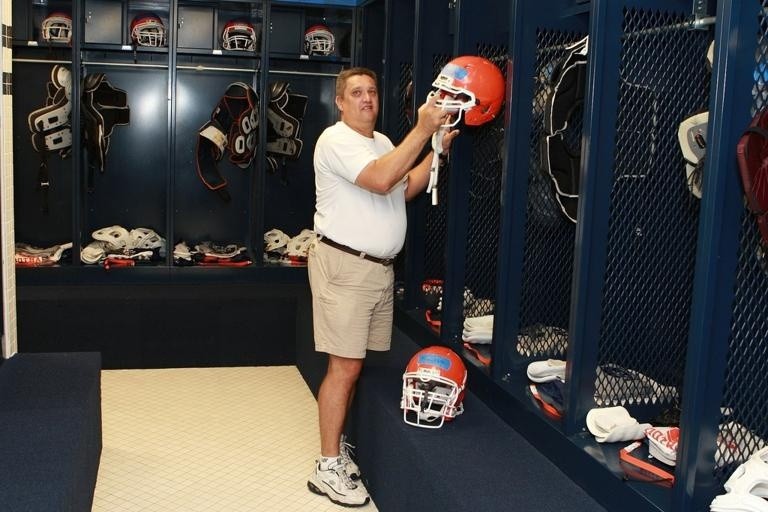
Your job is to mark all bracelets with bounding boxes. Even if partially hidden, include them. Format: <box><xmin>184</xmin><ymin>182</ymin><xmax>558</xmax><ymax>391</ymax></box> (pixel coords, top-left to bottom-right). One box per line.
<box><xmin>437</xmin><ymin>153</ymin><xmax>449</xmax><ymax>160</ymax></box>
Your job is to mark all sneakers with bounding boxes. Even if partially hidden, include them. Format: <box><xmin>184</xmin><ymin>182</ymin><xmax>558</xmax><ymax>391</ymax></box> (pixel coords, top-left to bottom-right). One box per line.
<box><xmin>340</xmin><ymin>435</ymin><xmax>361</xmax><ymax>480</ymax></box>
<box><xmin>307</xmin><ymin>460</ymin><xmax>371</xmax><ymax>507</ymax></box>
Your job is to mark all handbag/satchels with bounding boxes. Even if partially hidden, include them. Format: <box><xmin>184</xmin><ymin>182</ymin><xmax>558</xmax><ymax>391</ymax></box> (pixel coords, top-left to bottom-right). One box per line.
<box><xmin>643</xmin><ymin>423</ymin><xmax>766</xmax><ymax>470</ymax></box>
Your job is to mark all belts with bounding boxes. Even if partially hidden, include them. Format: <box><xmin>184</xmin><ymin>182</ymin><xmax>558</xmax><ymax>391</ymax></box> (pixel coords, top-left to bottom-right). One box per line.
<box><xmin>315</xmin><ymin>233</ymin><xmax>395</xmax><ymax>268</ymax></box>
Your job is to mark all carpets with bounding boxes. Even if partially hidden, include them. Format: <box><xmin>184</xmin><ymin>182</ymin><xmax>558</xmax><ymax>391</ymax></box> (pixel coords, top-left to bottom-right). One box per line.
<box><xmin>87</xmin><ymin>364</ymin><xmax>379</xmax><ymax>512</ymax></box>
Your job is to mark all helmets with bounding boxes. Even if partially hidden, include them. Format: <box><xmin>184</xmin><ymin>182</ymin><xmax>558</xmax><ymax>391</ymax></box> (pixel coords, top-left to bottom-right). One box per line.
<box><xmin>130</xmin><ymin>9</ymin><xmax>167</xmax><ymax>47</ymax></box>
<box><xmin>304</xmin><ymin>24</ymin><xmax>334</xmax><ymax>56</ymax></box>
<box><xmin>221</xmin><ymin>20</ymin><xmax>257</xmax><ymax>51</ymax></box>
<box><xmin>426</xmin><ymin>56</ymin><xmax>505</xmax><ymax>128</ymax></box>
<box><xmin>400</xmin><ymin>345</ymin><xmax>468</xmax><ymax>430</ymax></box>
<box><xmin>41</xmin><ymin>13</ymin><xmax>72</xmax><ymax>43</ymax></box>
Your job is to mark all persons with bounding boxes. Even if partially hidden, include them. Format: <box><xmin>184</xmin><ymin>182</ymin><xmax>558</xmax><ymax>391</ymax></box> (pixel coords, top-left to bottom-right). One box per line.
<box><xmin>303</xmin><ymin>64</ymin><xmax>460</xmax><ymax>508</ymax></box>
